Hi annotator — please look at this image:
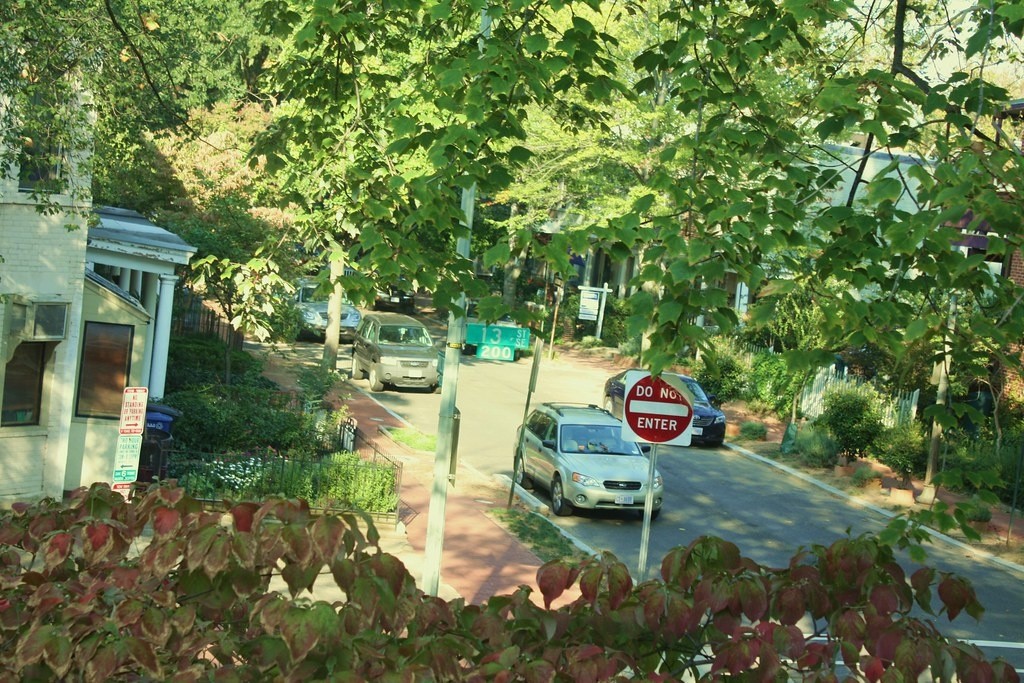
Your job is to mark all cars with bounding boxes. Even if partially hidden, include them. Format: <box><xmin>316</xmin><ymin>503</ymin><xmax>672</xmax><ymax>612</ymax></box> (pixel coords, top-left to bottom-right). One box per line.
<box><xmin>294</xmin><ymin>245</ymin><xmax>415</xmax><ymax>312</ymax></box>
<box><xmin>602</xmin><ymin>369</ymin><xmax>726</xmax><ymax>448</ymax></box>
<box><xmin>461</xmin><ymin>301</ymin><xmax>521</xmax><ymax>360</ymax></box>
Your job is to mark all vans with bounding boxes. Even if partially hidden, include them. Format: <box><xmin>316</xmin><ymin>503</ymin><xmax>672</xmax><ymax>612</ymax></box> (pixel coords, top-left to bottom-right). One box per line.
<box><xmin>351</xmin><ymin>314</ymin><xmax>438</xmax><ymax>391</ymax></box>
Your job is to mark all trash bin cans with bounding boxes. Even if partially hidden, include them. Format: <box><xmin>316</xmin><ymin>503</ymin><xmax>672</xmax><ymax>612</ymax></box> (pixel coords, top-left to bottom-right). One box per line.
<box><xmin>138</xmin><ymin>403</ymin><xmax>184</xmax><ymax>492</ymax></box>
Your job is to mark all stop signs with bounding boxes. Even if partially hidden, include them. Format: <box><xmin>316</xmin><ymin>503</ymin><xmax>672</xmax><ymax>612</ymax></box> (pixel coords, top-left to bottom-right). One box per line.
<box><xmin>621</xmin><ymin>371</ymin><xmax>694</xmax><ymax>447</ymax></box>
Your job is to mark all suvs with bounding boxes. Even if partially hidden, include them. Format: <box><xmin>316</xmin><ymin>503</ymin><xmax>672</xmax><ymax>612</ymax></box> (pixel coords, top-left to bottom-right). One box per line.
<box><xmin>289</xmin><ymin>278</ymin><xmax>362</xmax><ymax>341</ymax></box>
<box><xmin>513</xmin><ymin>401</ymin><xmax>663</xmax><ymax>521</ymax></box>
